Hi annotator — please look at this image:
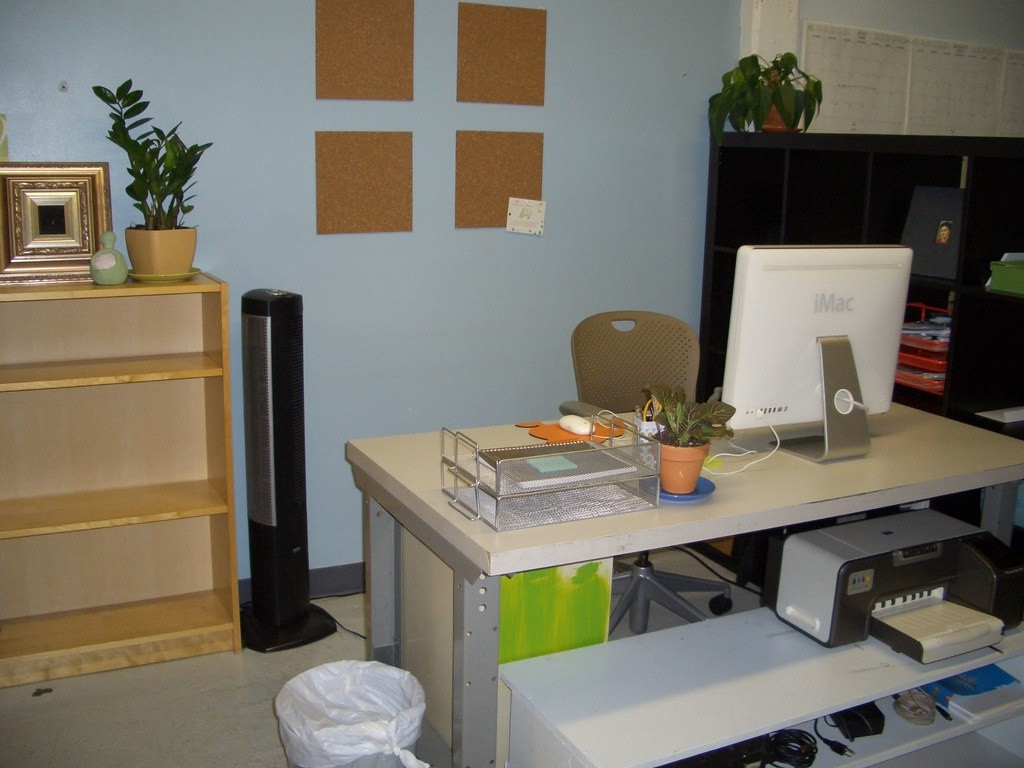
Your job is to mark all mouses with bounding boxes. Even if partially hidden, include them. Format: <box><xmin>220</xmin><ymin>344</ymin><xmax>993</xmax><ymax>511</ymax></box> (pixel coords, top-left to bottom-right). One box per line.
<box><xmin>560</xmin><ymin>413</ymin><xmax>595</xmax><ymax>435</ymax></box>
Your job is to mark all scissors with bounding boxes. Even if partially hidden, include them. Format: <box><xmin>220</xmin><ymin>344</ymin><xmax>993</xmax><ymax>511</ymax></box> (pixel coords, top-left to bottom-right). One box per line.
<box><xmin>643</xmin><ymin>397</ymin><xmax>662</xmax><ymax>422</ymax></box>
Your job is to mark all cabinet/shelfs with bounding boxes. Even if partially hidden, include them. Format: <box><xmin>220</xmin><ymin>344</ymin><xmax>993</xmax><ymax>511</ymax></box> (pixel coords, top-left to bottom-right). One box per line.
<box><xmin>0</xmin><ymin>269</ymin><xmax>244</xmax><ymax>690</ymax></box>
<box><xmin>498</xmin><ymin>608</ymin><xmax>1023</xmax><ymax>767</ymax></box>
<box><xmin>699</xmin><ymin>129</ymin><xmax>1024</xmax><ymax>439</ymax></box>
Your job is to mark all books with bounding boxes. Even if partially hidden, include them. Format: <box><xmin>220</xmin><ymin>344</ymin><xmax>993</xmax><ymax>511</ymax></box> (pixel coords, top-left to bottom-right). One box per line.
<box><xmin>921</xmin><ymin>664</ymin><xmax>1024</xmax><ymax>726</ymax></box>
<box><xmin>476</xmin><ymin>438</ymin><xmax>638</xmax><ymax>489</ymax></box>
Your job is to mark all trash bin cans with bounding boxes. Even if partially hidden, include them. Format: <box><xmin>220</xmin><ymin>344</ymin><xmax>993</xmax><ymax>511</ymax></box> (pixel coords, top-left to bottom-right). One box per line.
<box><xmin>274</xmin><ymin>659</ymin><xmax>426</xmax><ymax>768</ymax></box>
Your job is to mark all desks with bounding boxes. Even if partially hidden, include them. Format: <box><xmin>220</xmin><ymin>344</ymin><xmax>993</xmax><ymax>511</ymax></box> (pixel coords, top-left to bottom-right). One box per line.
<box><xmin>345</xmin><ymin>401</ymin><xmax>1024</xmax><ymax>768</ymax></box>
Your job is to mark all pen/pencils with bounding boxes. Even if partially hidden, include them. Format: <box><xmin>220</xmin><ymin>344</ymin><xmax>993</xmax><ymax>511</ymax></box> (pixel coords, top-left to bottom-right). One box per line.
<box><xmin>635</xmin><ymin>406</ymin><xmax>654</xmax><ymax>422</ymax></box>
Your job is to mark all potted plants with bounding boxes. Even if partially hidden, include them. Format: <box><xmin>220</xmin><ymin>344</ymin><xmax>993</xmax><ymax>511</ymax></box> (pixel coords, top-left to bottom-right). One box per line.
<box><xmin>638</xmin><ymin>385</ymin><xmax>737</xmax><ymax>493</ymax></box>
<box><xmin>88</xmin><ymin>80</ymin><xmax>214</xmax><ymax>282</ymax></box>
<box><xmin>704</xmin><ymin>51</ymin><xmax>822</xmax><ymax>146</ymax></box>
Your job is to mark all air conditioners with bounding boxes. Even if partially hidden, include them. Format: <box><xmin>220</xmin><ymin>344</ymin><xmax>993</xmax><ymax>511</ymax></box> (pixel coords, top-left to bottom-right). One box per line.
<box><xmin>233</xmin><ymin>287</ymin><xmax>339</xmax><ymax>652</ymax></box>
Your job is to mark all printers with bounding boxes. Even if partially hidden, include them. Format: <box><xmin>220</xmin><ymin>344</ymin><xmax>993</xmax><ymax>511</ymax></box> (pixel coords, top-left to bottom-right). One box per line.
<box><xmin>727</xmin><ymin>505</ymin><xmax>1023</xmax><ymax>664</ymax></box>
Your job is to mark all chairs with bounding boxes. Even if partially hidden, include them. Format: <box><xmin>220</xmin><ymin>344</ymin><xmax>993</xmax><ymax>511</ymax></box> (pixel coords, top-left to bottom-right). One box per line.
<box><xmin>558</xmin><ymin>310</ymin><xmax>735</xmax><ymax>638</ymax></box>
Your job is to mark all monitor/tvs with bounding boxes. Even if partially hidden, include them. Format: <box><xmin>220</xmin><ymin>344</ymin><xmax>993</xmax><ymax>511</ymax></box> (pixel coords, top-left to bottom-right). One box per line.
<box><xmin>725</xmin><ymin>243</ymin><xmax>914</xmax><ymax>465</ymax></box>
